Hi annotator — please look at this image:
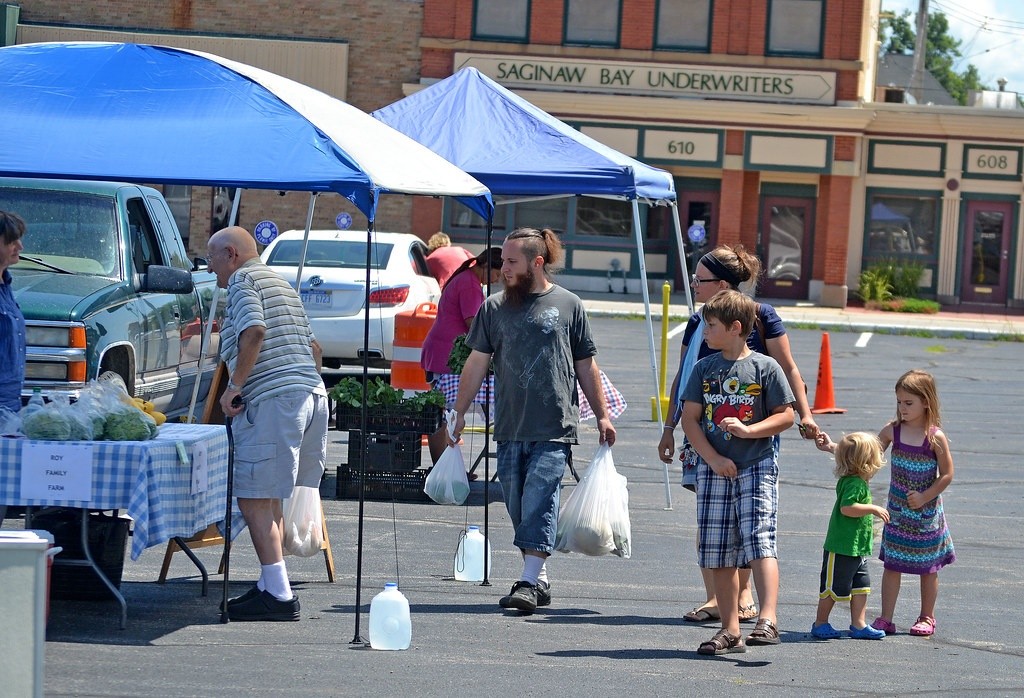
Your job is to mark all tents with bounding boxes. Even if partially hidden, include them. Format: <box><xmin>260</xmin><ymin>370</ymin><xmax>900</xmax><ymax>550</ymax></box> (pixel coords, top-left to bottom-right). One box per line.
<box><xmin>366</xmin><ymin>67</ymin><xmax>699</xmax><ymax>511</ymax></box>
<box><xmin>1</xmin><ymin>40</ymin><xmax>495</xmax><ymax>647</ymax></box>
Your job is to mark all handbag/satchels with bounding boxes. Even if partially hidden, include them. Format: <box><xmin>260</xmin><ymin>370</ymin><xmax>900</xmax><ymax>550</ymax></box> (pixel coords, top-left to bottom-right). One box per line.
<box><xmin>285</xmin><ymin>486</ymin><xmax>322</xmax><ymax>557</ymax></box>
<box><xmin>422</xmin><ymin>409</ymin><xmax>471</xmax><ymax>506</ymax></box>
<box><xmin>554</xmin><ymin>443</ymin><xmax>632</xmax><ymax>559</ymax></box>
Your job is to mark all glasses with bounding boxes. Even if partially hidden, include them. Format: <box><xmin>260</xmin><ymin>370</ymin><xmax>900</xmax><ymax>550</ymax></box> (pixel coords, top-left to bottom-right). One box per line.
<box><xmin>691</xmin><ymin>273</ymin><xmax>724</xmax><ymax>287</ymax></box>
<box><xmin>205</xmin><ymin>249</ymin><xmax>238</xmax><ymax>265</ymax></box>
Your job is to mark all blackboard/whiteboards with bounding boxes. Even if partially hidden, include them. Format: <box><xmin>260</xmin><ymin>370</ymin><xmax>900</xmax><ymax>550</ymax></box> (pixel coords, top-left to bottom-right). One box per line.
<box><xmin>169</xmin><ymin>360</ymin><xmax>333</xmax><ymax>551</ymax></box>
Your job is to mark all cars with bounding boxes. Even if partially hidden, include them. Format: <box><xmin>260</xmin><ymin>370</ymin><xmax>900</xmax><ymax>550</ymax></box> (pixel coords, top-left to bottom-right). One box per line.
<box><xmin>753</xmin><ymin>216</ymin><xmax>801</xmax><ymax>284</ymax></box>
<box><xmin>260</xmin><ymin>229</ymin><xmax>447</xmax><ymax>374</ymax></box>
<box><xmin>866</xmin><ymin>225</ymin><xmax>926</xmax><ymax>253</ymax></box>
<box><xmin>460</xmin><ymin>197</ymin><xmax>630</xmax><ymax>237</ymax></box>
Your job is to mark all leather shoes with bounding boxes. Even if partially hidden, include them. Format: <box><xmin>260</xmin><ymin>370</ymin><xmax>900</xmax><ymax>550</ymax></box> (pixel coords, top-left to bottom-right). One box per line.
<box><xmin>220</xmin><ymin>585</ymin><xmax>300</xmax><ymax>621</ymax></box>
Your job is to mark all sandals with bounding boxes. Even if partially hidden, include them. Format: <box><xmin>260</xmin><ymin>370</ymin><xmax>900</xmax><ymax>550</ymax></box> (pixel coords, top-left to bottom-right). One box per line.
<box><xmin>870</xmin><ymin>617</ymin><xmax>895</xmax><ymax>632</ymax></box>
<box><xmin>812</xmin><ymin>620</ymin><xmax>840</xmax><ymax>638</ymax></box>
<box><xmin>746</xmin><ymin>618</ymin><xmax>781</xmax><ymax>645</ymax></box>
<box><xmin>697</xmin><ymin>627</ymin><xmax>746</xmax><ymax>655</ymax></box>
<box><xmin>847</xmin><ymin>624</ymin><xmax>886</xmax><ymax>638</ymax></box>
<box><xmin>910</xmin><ymin>616</ymin><xmax>936</xmax><ymax>636</ymax></box>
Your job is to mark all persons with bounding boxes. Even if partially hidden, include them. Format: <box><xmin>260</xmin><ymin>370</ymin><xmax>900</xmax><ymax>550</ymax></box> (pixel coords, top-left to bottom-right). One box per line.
<box><xmin>204</xmin><ymin>225</ymin><xmax>329</xmax><ymax>621</ymax></box>
<box><xmin>679</xmin><ymin>287</ymin><xmax>796</xmax><ymax>655</ymax></box>
<box><xmin>420</xmin><ymin>245</ymin><xmax>504</xmax><ymax>390</ymax></box>
<box><xmin>810</xmin><ymin>430</ymin><xmax>891</xmax><ymax>639</ymax></box>
<box><xmin>657</xmin><ymin>242</ymin><xmax>820</xmax><ymax>622</ymax></box>
<box><xmin>817</xmin><ymin>370</ymin><xmax>953</xmax><ymax>636</ymax></box>
<box><xmin>446</xmin><ymin>227</ymin><xmax>616</xmax><ymax>612</ymax></box>
<box><xmin>0</xmin><ymin>212</ymin><xmax>28</xmax><ymax>414</ymax></box>
<box><xmin>425</xmin><ymin>229</ymin><xmax>476</xmax><ymax>290</ymax></box>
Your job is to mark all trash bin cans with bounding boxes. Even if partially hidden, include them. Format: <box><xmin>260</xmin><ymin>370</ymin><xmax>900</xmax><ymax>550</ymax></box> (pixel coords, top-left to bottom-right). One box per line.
<box><xmin>0</xmin><ymin>527</ymin><xmax>63</xmax><ymax>698</ymax></box>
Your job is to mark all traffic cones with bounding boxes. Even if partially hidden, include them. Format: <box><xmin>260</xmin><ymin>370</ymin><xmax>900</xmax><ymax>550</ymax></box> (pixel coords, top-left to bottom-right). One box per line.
<box><xmin>810</xmin><ymin>331</ymin><xmax>849</xmax><ymax>415</ymax></box>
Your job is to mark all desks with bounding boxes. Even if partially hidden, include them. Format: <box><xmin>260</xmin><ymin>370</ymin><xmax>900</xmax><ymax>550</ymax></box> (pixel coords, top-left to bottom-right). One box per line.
<box><xmin>0</xmin><ymin>423</ymin><xmax>247</xmax><ymax>631</ymax></box>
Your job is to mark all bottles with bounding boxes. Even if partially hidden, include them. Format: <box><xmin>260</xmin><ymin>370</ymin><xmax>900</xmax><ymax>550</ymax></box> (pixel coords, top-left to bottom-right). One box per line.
<box><xmin>369</xmin><ymin>583</ymin><xmax>412</xmax><ymax>650</ymax></box>
<box><xmin>28</xmin><ymin>387</ymin><xmax>45</xmax><ymax>413</ymax></box>
<box><xmin>454</xmin><ymin>526</ymin><xmax>490</xmax><ymax>582</ymax></box>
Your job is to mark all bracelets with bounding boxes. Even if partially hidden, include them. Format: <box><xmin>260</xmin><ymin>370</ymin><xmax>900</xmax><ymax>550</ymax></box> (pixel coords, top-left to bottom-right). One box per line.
<box><xmin>662</xmin><ymin>426</ymin><xmax>676</xmax><ymax>430</ymax></box>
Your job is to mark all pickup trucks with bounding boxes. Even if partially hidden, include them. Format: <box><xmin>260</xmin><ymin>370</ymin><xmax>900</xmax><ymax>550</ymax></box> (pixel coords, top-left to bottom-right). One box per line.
<box><xmin>0</xmin><ymin>173</ymin><xmax>232</xmax><ymax>423</ymax></box>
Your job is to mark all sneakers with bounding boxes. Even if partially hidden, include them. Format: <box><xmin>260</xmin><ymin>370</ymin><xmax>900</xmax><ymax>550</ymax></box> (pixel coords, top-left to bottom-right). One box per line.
<box><xmin>509</xmin><ymin>580</ymin><xmax>538</xmax><ymax>613</ymax></box>
<box><xmin>498</xmin><ymin>580</ymin><xmax>551</xmax><ymax>609</ymax></box>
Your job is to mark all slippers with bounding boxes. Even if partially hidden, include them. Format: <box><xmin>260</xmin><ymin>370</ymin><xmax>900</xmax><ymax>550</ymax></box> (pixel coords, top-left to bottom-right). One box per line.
<box><xmin>683</xmin><ymin>608</ymin><xmax>718</xmax><ymax>620</ymax></box>
<box><xmin>737</xmin><ymin>598</ymin><xmax>759</xmax><ymax>620</ymax></box>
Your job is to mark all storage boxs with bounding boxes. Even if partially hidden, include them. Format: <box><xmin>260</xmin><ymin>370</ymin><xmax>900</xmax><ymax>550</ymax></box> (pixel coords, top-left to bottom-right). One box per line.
<box><xmin>336</xmin><ymin>399</ymin><xmax>443</xmax><ymax>505</ymax></box>
